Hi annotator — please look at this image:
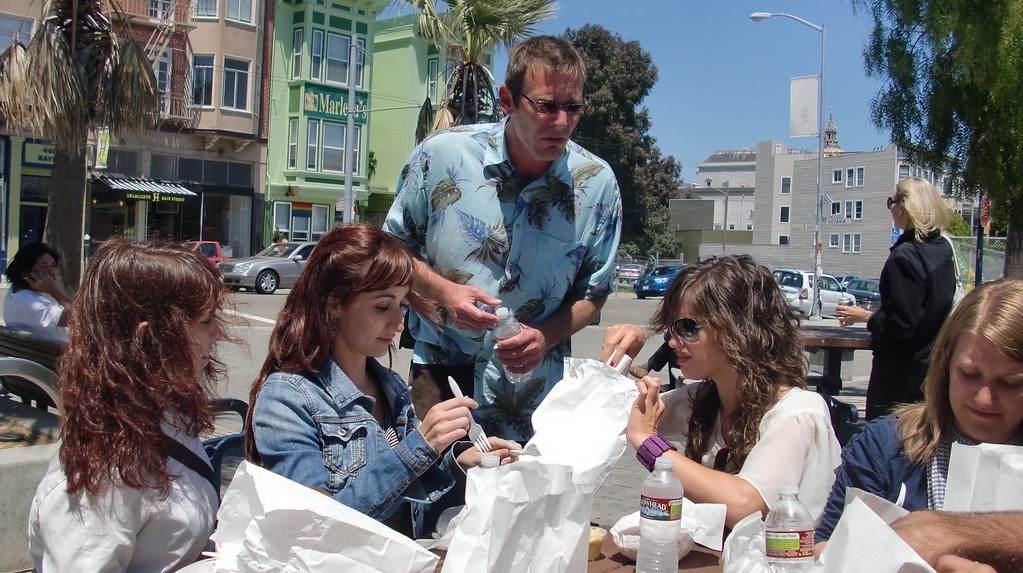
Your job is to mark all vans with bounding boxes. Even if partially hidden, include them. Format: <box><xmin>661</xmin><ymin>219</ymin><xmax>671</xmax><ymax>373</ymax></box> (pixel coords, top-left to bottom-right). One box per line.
<box><xmin>772</xmin><ymin>269</ymin><xmax>858</xmax><ymax>318</ymax></box>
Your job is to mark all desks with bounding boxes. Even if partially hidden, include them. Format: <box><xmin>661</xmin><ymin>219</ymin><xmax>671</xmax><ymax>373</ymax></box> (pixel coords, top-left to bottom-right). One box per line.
<box><xmin>792</xmin><ymin>323</ymin><xmax>872</xmax><ymax>423</ymax></box>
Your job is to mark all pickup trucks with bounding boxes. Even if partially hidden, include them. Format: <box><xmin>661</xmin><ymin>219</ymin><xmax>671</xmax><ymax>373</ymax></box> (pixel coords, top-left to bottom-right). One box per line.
<box><xmin>181</xmin><ymin>240</ymin><xmax>240</xmax><ymax>270</ymax></box>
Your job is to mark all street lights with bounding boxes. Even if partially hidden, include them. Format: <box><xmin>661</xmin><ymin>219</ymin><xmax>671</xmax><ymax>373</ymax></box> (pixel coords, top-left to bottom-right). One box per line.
<box><xmin>751</xmin><ymin>11</ymin><xmax>825</xmax><ymax>322</ymax></box>
<box><xmin>691</xmin><ymin>180</ymin><xmax>730</xmax><ymax>254</ymax></box>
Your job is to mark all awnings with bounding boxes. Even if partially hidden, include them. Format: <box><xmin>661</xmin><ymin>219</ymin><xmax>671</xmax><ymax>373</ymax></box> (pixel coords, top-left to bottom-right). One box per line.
<box><xmin>92</xmin><ymin>173</ymin><xmax>197</xmax><ymax>202</ymax></box>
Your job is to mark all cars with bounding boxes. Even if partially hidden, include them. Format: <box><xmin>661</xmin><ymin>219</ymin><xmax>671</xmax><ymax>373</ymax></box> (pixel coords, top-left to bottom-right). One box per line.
<box><xmin>829</xmin><ymin>274</ymin><xmax>857</xmax><ymax>290</ymax></box>
<box><xmin>634</xmin><ymin>262</ymin><xmax>690</xmax><ymax>300</ymax></box>
<box><xmin>214</xmin><ymin>241</ymin><xmax>318</xmax><ymax>295</ymax></box>
<box><xmin>618</xmin><ymin>263</ymin><xmax>646</xmax><ymax>284</ymax></box>
<box><xmin>845</xmin><ymin>279</ymin><xmax>882</xmax><ymax>313</ymax></box>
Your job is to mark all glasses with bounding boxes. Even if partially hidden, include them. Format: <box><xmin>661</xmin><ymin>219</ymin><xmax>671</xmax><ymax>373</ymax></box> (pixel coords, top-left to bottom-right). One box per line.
<box><xmin>510</xmin><ymin>88</ymin><xmax>588</xmax><ymax>116</ymax></box>
<box><xmin>669</xmin><ymin>317</ymin><xmax>712</xmax><ymax>345</ymax></box>
<box><xmin>887</xmin><ymin>197</ymin><xmax>902</xmax><ymax>208</ymax></box>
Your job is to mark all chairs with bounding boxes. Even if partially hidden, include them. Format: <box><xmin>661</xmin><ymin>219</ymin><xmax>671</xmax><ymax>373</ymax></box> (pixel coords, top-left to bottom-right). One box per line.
<box><xmin>192</xmin><ymin>398</ymin><xmax>254</xmax><ymax>510</ymax></box>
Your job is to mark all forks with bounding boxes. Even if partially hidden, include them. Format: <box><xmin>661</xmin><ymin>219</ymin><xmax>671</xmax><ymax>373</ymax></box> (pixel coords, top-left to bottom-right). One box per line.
<box><xmin>447</xmin><ymin>376</ymin><xmax>491</xmax><ymax>455</ymax></box>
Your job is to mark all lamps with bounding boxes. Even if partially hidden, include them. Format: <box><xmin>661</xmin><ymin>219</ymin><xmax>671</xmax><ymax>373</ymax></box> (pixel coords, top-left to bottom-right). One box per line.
<box><xmin>285</xmin><ymin>182</ymin><xmax>296</xmax><ymax>197</ymax></box>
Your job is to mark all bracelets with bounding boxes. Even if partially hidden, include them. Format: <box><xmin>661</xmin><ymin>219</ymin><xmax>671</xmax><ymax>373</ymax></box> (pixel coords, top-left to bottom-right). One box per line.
<box><xmin>636</xmin><ymin>433</ymin><xmax>679</xmax><ymax>472</ymax></box>
<box><xmin>866</xmin><ymin>315</ymin><xmax>871</xmax><ymax>321</ymax></box>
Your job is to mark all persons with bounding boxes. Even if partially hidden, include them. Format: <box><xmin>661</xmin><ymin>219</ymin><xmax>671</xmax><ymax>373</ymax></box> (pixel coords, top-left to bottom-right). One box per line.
<box><xmin>381</xmin><ymin>35</ymin><xmax>623</xmax><ymax>538</ymax></box>
<box><xmin>28</xmin><ymin>237</ymin><xmax>251</xmax><ymax>573</ymax></box>
<box><xmin>836</xmin><ymin>177</ymin><xmax>957</xmax><ymax>421</ymax></box>
<box><xmin>271</xmin><ymin>237</ymin><xmax>288</xmax><ymax>256</ymax></box>
<box><xmin>599</xmin><ymin>254</ymin><xmax>842</xmax><ymax>531</ymax></box>
<box><xmin>3</xmin><ymin>242</ymin><xmax>72</xmax><ymax>342</ymax></box>
<box><xmin>245</xmin><ymin>222</ymin><xmax>523</xmax><ymax>538</ymax></box>
<box><xmin>809</xmin><ymin>277</ymin><xmax>1023</xmax><ymax>573</ymax></box>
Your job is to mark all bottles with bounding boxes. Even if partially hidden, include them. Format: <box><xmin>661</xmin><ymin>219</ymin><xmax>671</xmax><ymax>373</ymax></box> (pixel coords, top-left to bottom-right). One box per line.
<box><xmin>495</xmin><ymin>306</ymin><xmax>532</xmax><ymax>384</ymax></box>
<box><xmin>635</xmin><ymin>457</ymin><xmax>684</xmax><ymax>573</ymax></box>
<box><xmin>763</xmin><ymin>484</ymin><xmax>816</xmax><ymax>572</ymax></box>
<box><xmin>480</xmin><ymin>454</ymin><xmax>501</xmax><ymax>470</ymax></box>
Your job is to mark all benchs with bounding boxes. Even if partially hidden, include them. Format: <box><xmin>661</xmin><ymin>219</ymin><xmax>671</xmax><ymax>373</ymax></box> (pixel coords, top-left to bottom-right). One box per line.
<box><xmin>0</xmin><ymin>325</ymin><xmax>67</xmax><ymax>411</ymax></box>
